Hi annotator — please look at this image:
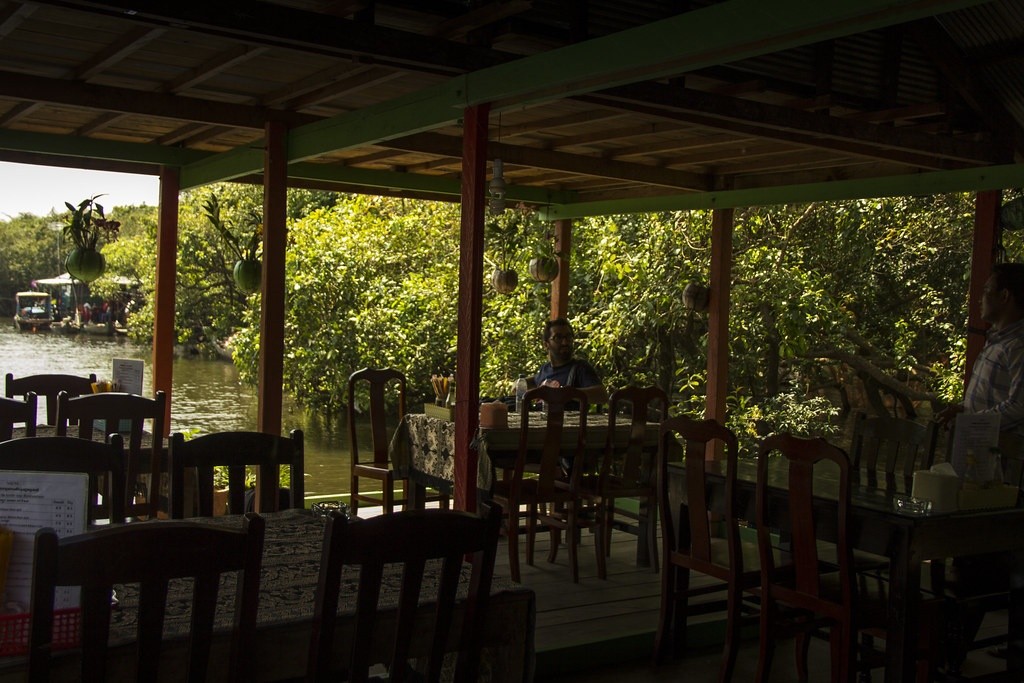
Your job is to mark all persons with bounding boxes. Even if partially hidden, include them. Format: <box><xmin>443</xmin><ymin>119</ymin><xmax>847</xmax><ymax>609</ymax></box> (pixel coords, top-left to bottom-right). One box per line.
<box><xmin>35</xmin><ymin>297</ymin><xmax>44</xmax><ymax>306</ymax></box>
<box><xmin>84</xmin><ymin>300</ymin><xmax>109</xmax><ymax>323</ymax></box>
<box><xmin>511</xmin><ymin>319</ymin><xmax>607</xmax><ymax>412</ymax></box>
<box><xmin>934</xmin><ymin>263</ymin><xmax>1024</xmax><ymax>486</ymax></box>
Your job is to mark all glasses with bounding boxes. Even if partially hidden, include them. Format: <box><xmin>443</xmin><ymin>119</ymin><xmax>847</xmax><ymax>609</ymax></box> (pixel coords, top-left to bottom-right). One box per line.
<box><xmin>549</xmin><ymin>331</ymin><xmax>577</xmax><ymax>344</ymax></box>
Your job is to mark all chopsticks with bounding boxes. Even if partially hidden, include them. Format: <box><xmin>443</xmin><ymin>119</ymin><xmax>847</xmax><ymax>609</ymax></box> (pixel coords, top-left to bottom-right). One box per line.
<box><xmin>431</xmin><ymin>375</ymin><xmax>454</xmax><ymax>398</ymax></box>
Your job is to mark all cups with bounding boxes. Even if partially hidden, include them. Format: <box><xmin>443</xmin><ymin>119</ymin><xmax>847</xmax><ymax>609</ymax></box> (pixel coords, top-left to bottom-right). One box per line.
<box><xmin>435</xmin><ymin>398</ymin><xmax>445</xmax><ymax>407</ymax></box>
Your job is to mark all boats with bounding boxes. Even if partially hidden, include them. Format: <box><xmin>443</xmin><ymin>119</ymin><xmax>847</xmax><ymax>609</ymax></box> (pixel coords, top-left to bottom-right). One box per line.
<box><xmin>12</xmin><ymin>290</ymin><xmax>55</xmax><ymax>332</ymax></box>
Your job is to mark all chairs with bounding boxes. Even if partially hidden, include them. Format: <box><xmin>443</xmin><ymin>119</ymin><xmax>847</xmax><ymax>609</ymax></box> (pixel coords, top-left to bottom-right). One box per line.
<box><xmin>0</xmin><ymin>366</ymin><xmax>1024</xmax><ymax>683</ymax></box>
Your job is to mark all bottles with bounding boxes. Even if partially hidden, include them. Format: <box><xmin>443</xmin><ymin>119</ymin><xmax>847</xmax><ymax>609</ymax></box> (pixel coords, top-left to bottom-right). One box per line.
<box><xmin>445</xmin><ymin>381</ymin><xmax>456</xmax><ymax>410</ymax></box>
<box><xmin>515</xmin><ymin>374</ymin><xmax>528</xmax><ymax>413</ymax></box>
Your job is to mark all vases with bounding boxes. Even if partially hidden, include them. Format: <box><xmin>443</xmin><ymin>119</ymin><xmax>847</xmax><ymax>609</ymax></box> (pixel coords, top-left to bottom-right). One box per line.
<box><xmin>65</xmin><ymin>249</ymin><xmax>106</xmax><ymax>283</ymax></box>
<box><xmin>233</xmin><ymin>260</ymin><xmax>262</xmax><ymax>295</ymax></box>
<box><xmin>529</xmin><ymin>256</ymin><xmax>559</xmax><ymax>282</ymax></box>
<box><xmin>681</xmin><ymin>283</ymin><xmax>710</xmax><ymax>313</ymax></box>
<box><xmin>493</xmin><ymin>270</ymin><xmax>518</xmax><ymax>295</ymax></box>
<box><xmin>1000</xmin><ymin>197</ymin><xmax>1024</xmax><ymax>230</ymax></box>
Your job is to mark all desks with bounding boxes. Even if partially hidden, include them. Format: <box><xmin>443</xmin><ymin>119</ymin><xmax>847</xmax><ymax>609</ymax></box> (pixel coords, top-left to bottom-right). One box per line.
<box><xmin>0</xmin><ymin>509</ymin><xmax>537</xmax><ymax>683</ymax></box>
<box><xmin>652</xmin><ymin>460</ymin><xmax>1024</xmax><ymax>683</ymax></box>
<box><xmin>10</xmin><ymin>426</ymin><xmax>169</xmax><ymax>520</ymax></box>
<box><xmin>388</xmin><ymin>411</ymin><xmax>662</xmax><ymax>568</ymax></box>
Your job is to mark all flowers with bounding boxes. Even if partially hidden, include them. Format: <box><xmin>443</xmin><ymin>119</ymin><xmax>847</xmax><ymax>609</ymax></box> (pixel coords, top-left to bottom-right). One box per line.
<box><xmin>62</xmin><ymin>194</ymin><xmax>121</xmax><ymax>253</ymax></box>
<box><xmin>200</xmin><ymin>193</ymin><xmax>268</xmax><ymax>262</ymax></box>
<box><xmin>486</xmin><ymin>202</ymin><xmax>556</xmax><ymax>272</ymax></box>
